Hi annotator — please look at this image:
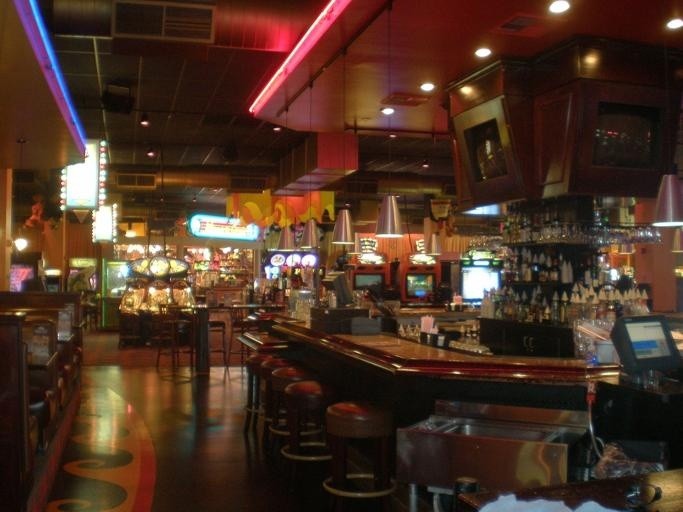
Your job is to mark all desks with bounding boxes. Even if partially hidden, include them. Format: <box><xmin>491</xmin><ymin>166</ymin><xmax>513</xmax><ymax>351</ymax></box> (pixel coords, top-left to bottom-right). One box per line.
<box><xmin>235</xmin><ymin>330</ymin><xmax>300</xmax><ymax>402</ymax></box>
<box><xmin>457</xmin><ymin>469</ymin><xmax>683</xmax><ymax>511</ymax></box>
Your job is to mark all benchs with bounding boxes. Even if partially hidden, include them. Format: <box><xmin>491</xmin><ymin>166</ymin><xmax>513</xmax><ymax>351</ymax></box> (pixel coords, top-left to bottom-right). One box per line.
<box><xmin>0</xmin><ymin>292</ymin><xmax>84</xmax><ymax>511</ymax></box>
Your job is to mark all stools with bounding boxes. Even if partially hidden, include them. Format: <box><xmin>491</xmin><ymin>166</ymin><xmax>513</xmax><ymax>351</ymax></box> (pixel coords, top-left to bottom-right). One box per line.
<box><xmin>208</xmin><ymin>321</ymin><xmax>232</xmax><ymax>380</ymax></box>
<box><xmin>240</xmin><ymin>318</ymin><xmax>262</xmax><ymax>364</ymax></box>
<box><xmin>244</xmin><ymin>353</ymin><xmax>399</xmax><ymax>512</ymax></box>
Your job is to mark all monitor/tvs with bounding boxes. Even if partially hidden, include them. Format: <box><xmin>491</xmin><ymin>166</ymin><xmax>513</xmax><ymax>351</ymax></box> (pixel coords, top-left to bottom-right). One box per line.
<box><xmin>460</xmin><ymin>259</ymin><xmax>501</xmax><ymax>307</ymax></box>
<box><xmin>610</xmin><ymin>313</ymin><xmax>675</xmax><ymax>374</ymax></box>
<box><xmin>9</xmin><ymin>264</ymin><xmax>36</xmax><ymax>294</ymax></box>
<box><xmin>667</xmin><ymin>317</ymin><xmax>683</xmax><ymax>362</ymax></box>
<box><xmin>321</xmin><ymin>271</ymin><xmax>354</xmax><ymax>304</ymax></box>
<box><xmin>461</xmin><ymin>118</ymin><xmax>511</xmax><ymax>183</ymax></box>
<box><xmin>592</xmin><ymin>122</ymin><xmax>658</xmax><ymax>172</ymax></box>
<box><xmin>354</xmin><ymin>272</ymin><xmax>385</xmax><ymax>290</ymax></box>
<box><xmin>405</xmin><ymin>273</ymin><xmax>436</xmax><ymax>299</ymax></box>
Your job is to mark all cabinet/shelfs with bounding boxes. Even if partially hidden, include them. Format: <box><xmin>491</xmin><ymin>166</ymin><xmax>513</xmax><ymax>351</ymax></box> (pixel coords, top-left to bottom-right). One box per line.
<box><xmin>182</xmin><ymin>238</ymin><xmax>263</xmax><ymax>301</ymax></box>
<box><xmin>475</xmin><ymin>239</ymin><xmax>614</xmax><ymax>357</ymax></box>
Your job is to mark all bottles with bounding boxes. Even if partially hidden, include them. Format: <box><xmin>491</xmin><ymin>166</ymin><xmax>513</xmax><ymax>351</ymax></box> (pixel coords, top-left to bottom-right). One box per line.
<box><xmin>251</xmin><ymin>270</ymin><xmax>290</xmax><ymax>304</ymax></box>
<box><xmin>481</xmin><ymin>287</ymin><xmax>650</xmax><ymax>324</ymax></box>
<box><xmin>497</xmin><ymin>212</ymin><xmax>661</xmax><ymax>244</ymax></box>
<box><xmin>497</xmin><ymin>247</ymin><xmax>635</xmax><ymax>286</ymax></box>
<box><xmin>568</xmin><ymin>325</ymin><xmax>599</xmax><ymax>366</ymax></box>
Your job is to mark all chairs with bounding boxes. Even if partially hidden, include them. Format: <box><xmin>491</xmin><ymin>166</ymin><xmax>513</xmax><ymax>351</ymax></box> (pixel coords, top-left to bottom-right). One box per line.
<box><xmin>156</xmin><ymin>303</ymin><xmax>195</xmax><ymax>371</ymax></box>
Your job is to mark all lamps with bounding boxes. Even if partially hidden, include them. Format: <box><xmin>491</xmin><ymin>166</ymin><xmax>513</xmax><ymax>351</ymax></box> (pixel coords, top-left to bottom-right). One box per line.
<box><xmin>299</xmin><ymin>84</ymin><xmax>321</xmax><ymax>249</ymax></box>
<box><xmin>374</xmin><ymin>3</ymin><xmax>404</xmax><ymax>239</ymax></box>
<box><xmin>617</xmin><ymin>0</ymin><xmax>683</xmax><ymax>253</ymax></box>
<box><xmin>139</xmin><ymin>112</ymin><xmax>149</xmax><ymax>126</ymax></box>
<box><xmin>420</xmin><ymin>83</ymin><xmax>435</xmax><ymax>91</ymax></box>
<box><xmin>330</xmin><ymin>50</ymin><xmax>354</xmax><ymax>245</ymax></box>
<box><xmin>276</xmin><ymin>108</ymin><xmax>298</xmax><ymax>251</ymax></box>
<box><xmin>147</xmin><ymin>149</ymin><xmax>154</xmax><ymax>156</ymax></box>
